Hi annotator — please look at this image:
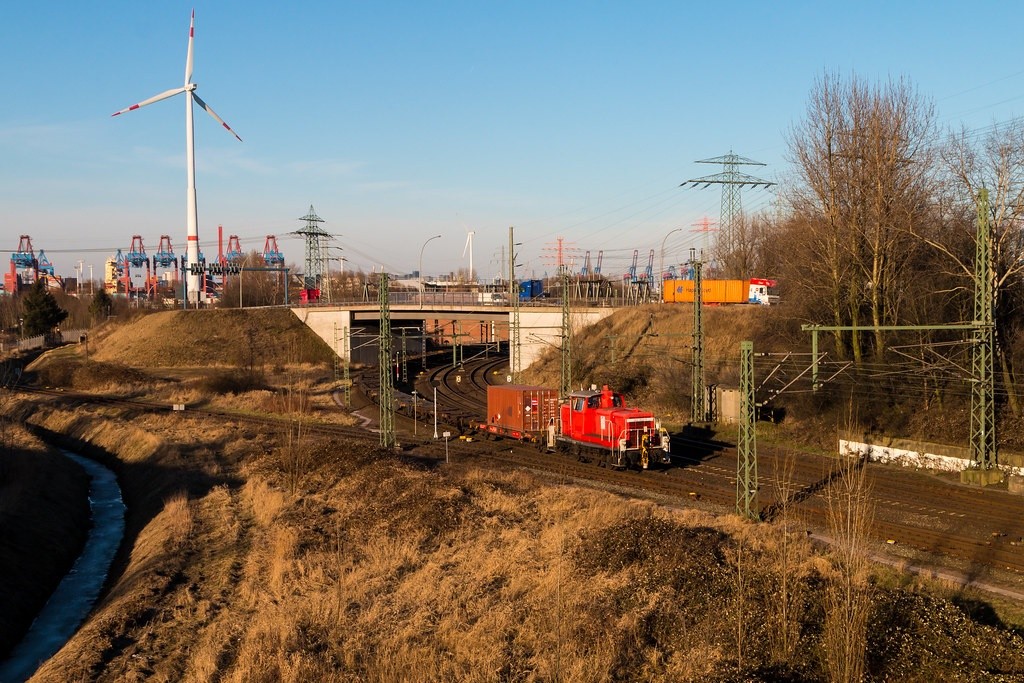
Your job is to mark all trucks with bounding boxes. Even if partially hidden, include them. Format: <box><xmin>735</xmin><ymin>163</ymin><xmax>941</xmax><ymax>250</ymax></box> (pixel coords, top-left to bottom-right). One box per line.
<box><xmin>518</xmin><ymin>279</ymin><xmax>551</xmax><ymax>302</ymax></box>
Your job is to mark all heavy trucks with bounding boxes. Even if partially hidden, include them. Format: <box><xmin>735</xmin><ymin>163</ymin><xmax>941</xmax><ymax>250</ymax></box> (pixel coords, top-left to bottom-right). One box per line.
<box><xmin>664</xmin><ymin>279</ymin><xmax>779</xmax><ymax>304</ymax></box>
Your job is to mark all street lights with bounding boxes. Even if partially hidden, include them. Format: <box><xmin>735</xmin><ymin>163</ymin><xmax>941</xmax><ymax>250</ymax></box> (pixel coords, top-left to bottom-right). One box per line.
<box><xmin>419</xmin><ymin>235</ymin><xmax>442</xmax><ymax>306</ymax></box>
<box><xmin>239</xmin><ymin>251</ymin><xmax>262</xmax><ymax>309</ymax></box>
<box><xmin>148</xmin><ymin>281</ymin><xmax>163</xmax><ymax>298</ymax></box>
<box><xmin>659</xmin><ymin>229</ymin><xmax>682</xmax><ymax>308</ymax></box>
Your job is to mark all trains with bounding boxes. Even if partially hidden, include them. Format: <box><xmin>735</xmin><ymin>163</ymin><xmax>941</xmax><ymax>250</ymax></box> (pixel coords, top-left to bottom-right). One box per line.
<box><xmin>483</xmin><ymin>383</ymin><xmax>672</xmax><ymax>467</ymax></box>
